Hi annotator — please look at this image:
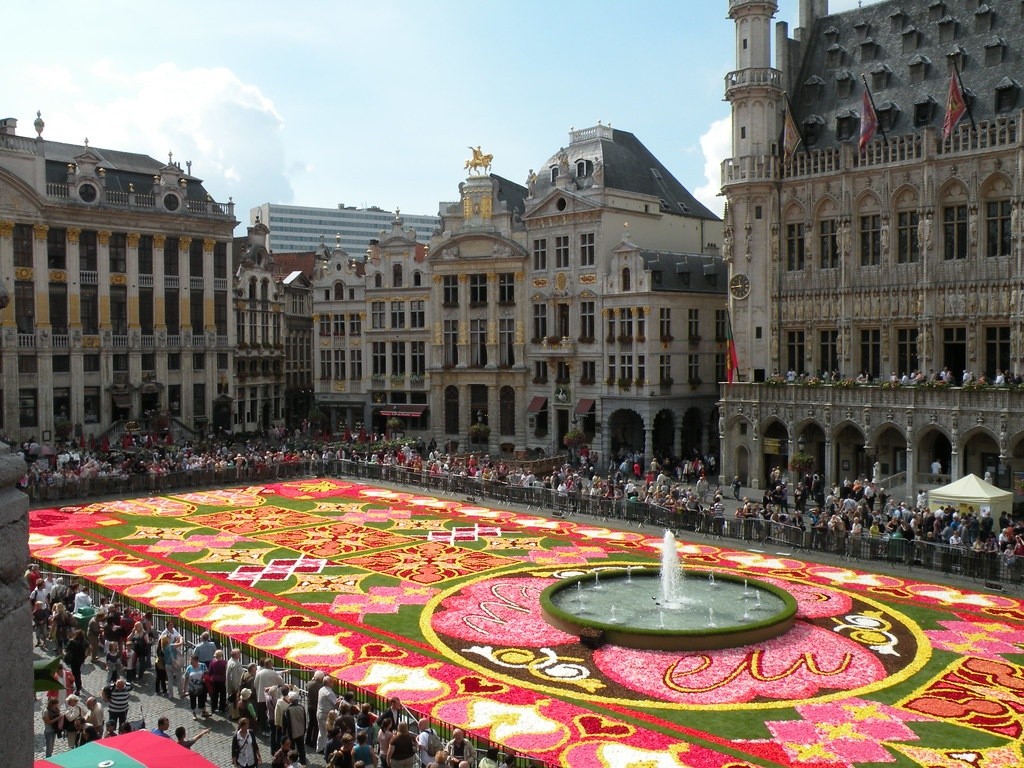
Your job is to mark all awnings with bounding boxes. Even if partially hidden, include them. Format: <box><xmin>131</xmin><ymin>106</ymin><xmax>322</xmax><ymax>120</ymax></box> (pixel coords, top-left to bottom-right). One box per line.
<box><xmin>379</xmin><ymin>405</ymin><xmax>428</xmax><ymax>417</ymax></box>
<box><xmin>574</xmin><ymin>400</ymin><xmax>595</xmax><ymax>417</ymax></box>
<box><xmin>527</xmin><ymin>396</ymin><xmax>547</xmax><ymax>415</ymax></box>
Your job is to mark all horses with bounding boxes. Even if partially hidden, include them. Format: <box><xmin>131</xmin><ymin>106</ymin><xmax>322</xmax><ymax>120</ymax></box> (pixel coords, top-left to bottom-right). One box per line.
<box><xmin>463</xmin><ymin>154</ymin><xmax>493</xmax><ymax>175</ymax></box>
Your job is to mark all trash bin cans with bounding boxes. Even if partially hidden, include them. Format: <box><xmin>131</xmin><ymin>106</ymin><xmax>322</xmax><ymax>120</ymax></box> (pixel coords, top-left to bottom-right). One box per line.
<box><xmin>628</xmin><ymin>496</ymin><xmax>641</xmax><ymax>520</ymax></box>
<box><xmin>889</xmin><ymin>532</ymin><xmax>903</xmax><ymax>562</ymax></box>
<box><xmin>227</xmin><ymin>463</ymin><xmax>236</xmax><ymax>482</ymax></box>
<box><xmin>369</xmin><ymin>459</ymin><xmax>379</xmax><ymax>478</ymax></box>
<box><xmin>73</xmin><ymin>605</ymin><xmax>98</xmax><ymax>638</ymax></box>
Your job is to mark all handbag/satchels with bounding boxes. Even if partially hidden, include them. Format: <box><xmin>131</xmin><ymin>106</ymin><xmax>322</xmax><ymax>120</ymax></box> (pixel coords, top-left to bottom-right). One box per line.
<box><xmin>58</xmin><ymin>715</ymin><xmax>65</xmax><ymax>731</ymax></box>
<box><xmin>409</xmin><ymin>733</ymin><xmax>418</xmax><ymax>753</ymax></box>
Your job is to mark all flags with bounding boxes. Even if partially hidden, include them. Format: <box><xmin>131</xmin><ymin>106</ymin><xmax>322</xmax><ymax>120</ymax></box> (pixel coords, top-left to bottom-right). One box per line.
<box><xmin>726</xmin><ymin>315</ymin><xmax>738</xmax><ymax>386</ymax></box>
<box><xmin>858</xmin><ymin>89</ymin><xmax>879</xmax><ymax>154</ymax></box>
<box><xmin>941</xmin><ymin>63</ymin><xmax>968</xmax><ymax>143</ymax></box>
<box><xmin>783</xmin><ymin>99</ymin><xmax>803</xmax><ymax>169</ymax></box>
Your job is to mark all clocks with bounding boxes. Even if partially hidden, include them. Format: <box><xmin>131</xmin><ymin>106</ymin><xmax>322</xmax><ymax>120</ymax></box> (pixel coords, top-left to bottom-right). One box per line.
<box><xmin>728</xmin><ymin>272</ymin><xmax>752</xmax><ymax>300</ymax></box>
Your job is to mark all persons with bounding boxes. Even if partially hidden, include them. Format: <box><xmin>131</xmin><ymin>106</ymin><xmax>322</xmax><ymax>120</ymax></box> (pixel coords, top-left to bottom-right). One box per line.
<box><xmin>232</xmin><ymin>657</ymin><xmax>543</xmax><ymax>768</ymax></box>
<box><xmin>151</xmin><ymin>716</ymin><xmax>212</xmax><ymax>750</ymax></box>
<box><xmin>24</xmin><ymin>564</ymin><xmax>153</xmax><ymax>696</ymax></box>
<box><xmin>722</xmin><ymin>204</ymin><xmax>1024</xmax><ymax>264</ymax></box>
<box><xmin>526</xmin><ymin>169</ymin><xmax>536</xmax><ymax>198</ymax></box>
<box><xmin>155</xmin><ymin>620</ymin><xmax>244</xmax><ymax>722</ymax></box>
<box><xmin>728</xmin><ymin>465</ymin><xmax>1024</xmax><ymax>584</ymax></box>
<box><xmin>469</xmin><ymin>146</ymin><xmax>484</xmax><ymax>161</ymax></box>
<box><xmin>477</xmin><ymin>408</ymin><xmax>485</xmax><ymax>422</ymax></box>
<box><xmin>42</xmin><ymin>664</ymin><xmax>134</xmax><ymax>758</ymax></box>
<box><xmin>591</xmin><ymin>157</ymin><xmax>602</xmax><ymax>185</ymax></box>
<box><xmin>771</xmin><ymin>367</ymin><xmax>1024</xmax><ymax>387</ymax></box>
<box><xmin>558</xmin><ymin>147</ymin><xmax>569</xmax><ymax>175</ymax></box>
<box><xmin>0</xmin><ymin>419</ymin><xmax>336</xmax><ymax>503</ymax></box>
<box><xmin>542</xmin><ymin>440</ymin><xmax>726</xmax><ymax>535</ymax></box>
<box><xmin>337</xmin><ymin>427</ymin><xmax>541</xmax><ymax>503</ymax></box>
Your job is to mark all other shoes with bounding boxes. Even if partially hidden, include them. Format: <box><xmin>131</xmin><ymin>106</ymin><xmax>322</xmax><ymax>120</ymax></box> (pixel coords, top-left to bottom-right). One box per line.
<box><xmin>194</xmin><ymin>714</ymin><xmax>198</xmax><ymax>721</ymax></box>
<box><xmin>180</xmin><ymin>692</ymin><xmax>189</xmax><ymax>697</ymax></box>
<box><xmin>202</xmin><ymin>711</ymin><xmax>213</xmax><ymax>716</ymax></box>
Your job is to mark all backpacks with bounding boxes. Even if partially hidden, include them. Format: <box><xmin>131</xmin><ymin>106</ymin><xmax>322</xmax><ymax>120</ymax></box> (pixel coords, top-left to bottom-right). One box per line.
<box><xmin>421</xmin><ymin>729</ymin><xmax>444</xmax><ymax>757</ymax></box>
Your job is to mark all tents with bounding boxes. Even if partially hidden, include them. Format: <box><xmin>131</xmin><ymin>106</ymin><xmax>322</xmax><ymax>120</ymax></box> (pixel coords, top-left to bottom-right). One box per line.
<box><xmin>928</xmin><ymin>473</ymin><xmax>1014</xmax><ymax>540</ymax></box>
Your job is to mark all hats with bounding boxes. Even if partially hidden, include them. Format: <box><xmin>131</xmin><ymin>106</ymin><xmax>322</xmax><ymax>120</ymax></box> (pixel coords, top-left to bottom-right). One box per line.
<box><xmin>505</xmin><ymin>754</ymin><xmax>514</xmax><ymax>765</ymax></box>
<box><xmin>65</xmin><ymin>694</ymin><xmax>78</xmax><ymax>701</ymax></box>
<box><xmin>239</xmin><ymin>688</ymin><xmax>252</xmax><ymax>700</ymax></box>
<box><xmin>288</xmin><ymin>691</ymin><xmax>299</xmax><ymax>701</ymax></box>
